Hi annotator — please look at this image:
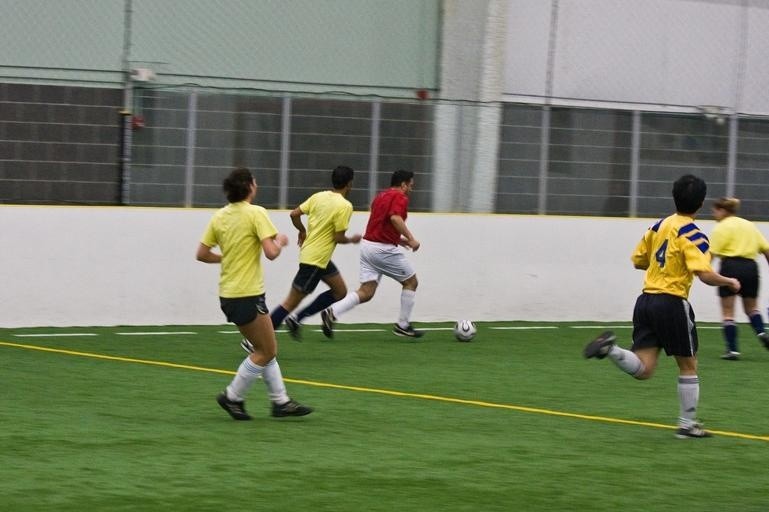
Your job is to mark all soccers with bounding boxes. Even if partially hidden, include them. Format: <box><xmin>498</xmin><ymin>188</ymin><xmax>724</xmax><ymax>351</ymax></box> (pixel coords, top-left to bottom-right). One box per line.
<box><xmin>455</xmin><ymin>320</ymin><xmax>476</xmax><ymax>341</ymax></box>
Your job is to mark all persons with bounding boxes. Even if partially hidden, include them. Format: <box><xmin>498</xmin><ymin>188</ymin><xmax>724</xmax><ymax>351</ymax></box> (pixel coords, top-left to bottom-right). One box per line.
<box><xmin>710</xmin><ymin>194</ymin><xmax>769</xmax><ymax>360</ymax></box>
<box><xmin>238</xmin><ymin>166</ymin><xmax>362</xmax><ymax>352</ymax></box>
<box><xmin>581</xmin><ymin>174</ymin><xmax>741</xmax><ymax>441</ymax></box>
<box><xmin>196</xmin><ymin>168</ymin><xmax>313</xmax><ymax>420</ymax></box>
<box><xmin>321</xmin><ymin>169</ymin><xmax>424</xmax><ymax>338</ymax></box>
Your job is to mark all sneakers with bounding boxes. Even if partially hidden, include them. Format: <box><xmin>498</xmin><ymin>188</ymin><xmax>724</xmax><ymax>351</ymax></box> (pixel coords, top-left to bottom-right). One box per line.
<box><xmin>392</xmin><ymin>323</ymin><xmax>422</xmax><ymax>338</ymax></box>
<box><xmin>720</xmin><ymin>351</ymin><xmax>740</xmax><ymax>360</ymax></box>
<box><xmin>240</xmin><ymin>339</ymin><xmax>254</xmax><ymax>354</ymax></box>
<box><xmin>321</xmin><ymin>308</ymin><xmax>336</xmax><ymax>339</ymax></box>
<box><xmin>584</xmin><ymin>331</ymin><xmax>617</xmax><ymax>360</ymax></box>
<box><xmin>271</xmin><ymin>398</ymin><xmax>312</xmax><ymax>418</ymax></box>
<box><xmin>216</xmin><ymin>391</ymin><xmax>249</xmax><ymax>420</ymax></box>
<box><xmin>284</xmin><ymin>315</ymin><xmax>304</xmax><ymax>342</ymax></box>
<box><xmin>674</xmin><ymin>425</ymin><xmax>712</xmax><ymax>439</ymax></box>
